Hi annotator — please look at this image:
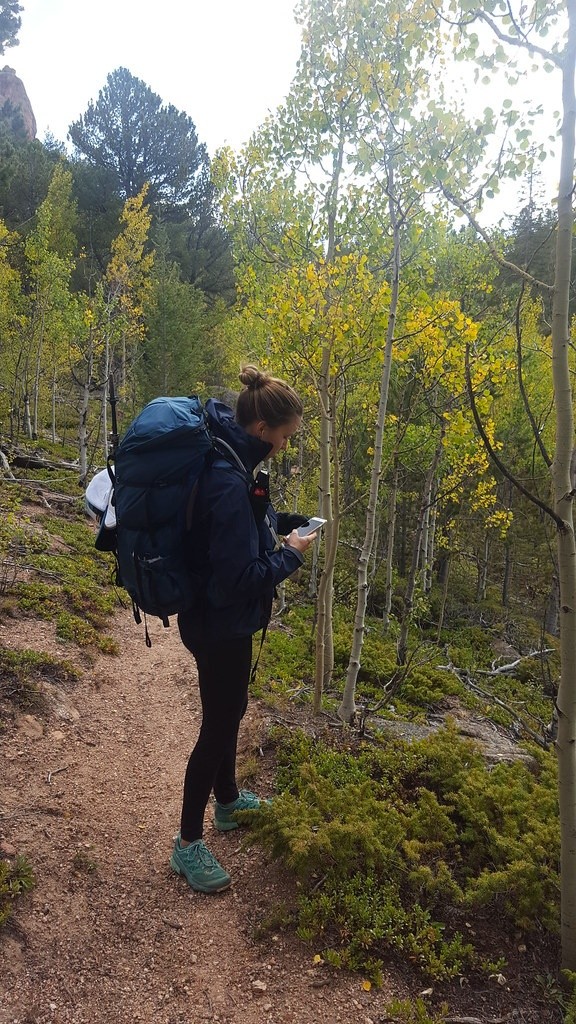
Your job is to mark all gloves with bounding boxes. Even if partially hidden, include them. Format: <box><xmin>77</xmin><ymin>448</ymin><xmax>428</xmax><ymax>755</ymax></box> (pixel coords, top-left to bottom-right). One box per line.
<box><xmin>276</xmin><ymin>512</ymin><xmax>310</xmax><ymax>535</ymax></box>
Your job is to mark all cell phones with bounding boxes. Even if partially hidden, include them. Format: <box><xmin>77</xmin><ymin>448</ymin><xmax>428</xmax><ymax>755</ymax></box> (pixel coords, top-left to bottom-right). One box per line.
<box><xmin>287</xmin><ymin>517</ymin><xmax>327</xmax><ymax>538</ymax></box>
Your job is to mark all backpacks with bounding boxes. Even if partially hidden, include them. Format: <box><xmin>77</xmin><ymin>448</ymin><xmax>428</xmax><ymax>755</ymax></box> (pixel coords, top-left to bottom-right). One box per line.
<box><xmin>85</xmin><ymin>396</ymin><xmax>254</xmax><ymax>616</ymax></box>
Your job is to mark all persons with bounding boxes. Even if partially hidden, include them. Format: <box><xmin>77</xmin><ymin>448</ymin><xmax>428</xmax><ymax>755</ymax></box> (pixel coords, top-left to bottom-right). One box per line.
<box><xmin>168</xmin><ymin>362</ymin><xmax>319</xmax><ymax>891</ymax></box>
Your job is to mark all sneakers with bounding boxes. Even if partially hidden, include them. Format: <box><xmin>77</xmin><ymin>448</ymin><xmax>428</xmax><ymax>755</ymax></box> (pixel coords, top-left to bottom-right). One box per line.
<box><xmin>214</xmin><ymin>791</ymin><xmax>274</xmax><ymax>830</ymax></box>
<box><xmin>170</xmin><ymin>837</ymin><xmax>231</xmax><ymax>894</ymax></box>
